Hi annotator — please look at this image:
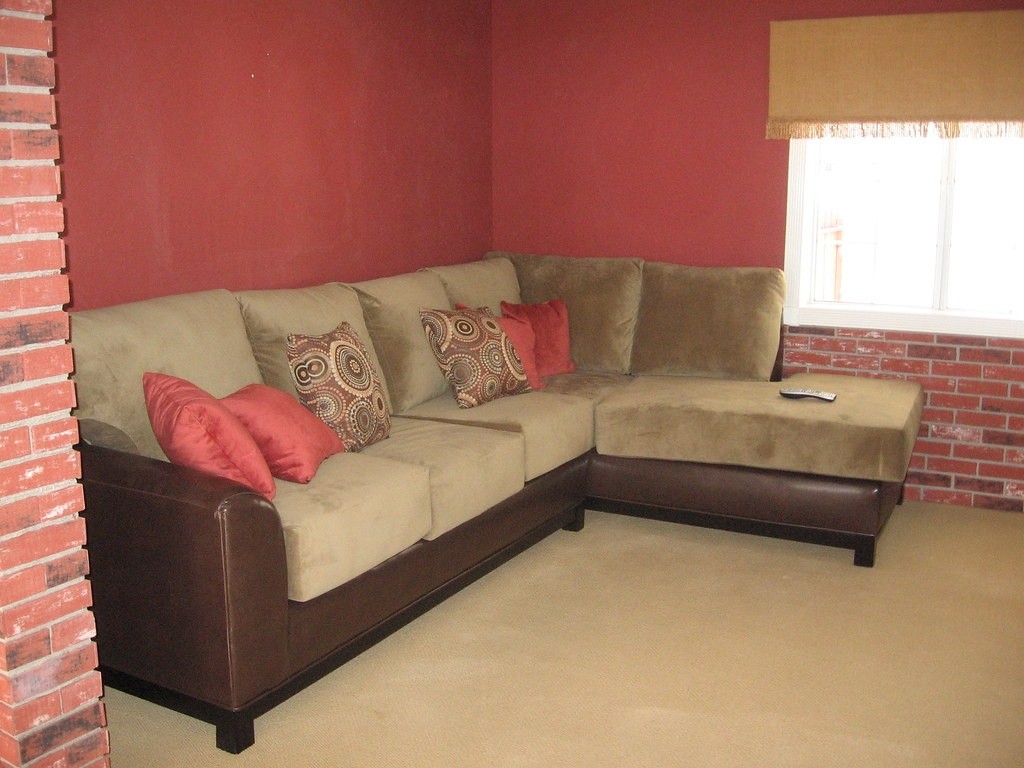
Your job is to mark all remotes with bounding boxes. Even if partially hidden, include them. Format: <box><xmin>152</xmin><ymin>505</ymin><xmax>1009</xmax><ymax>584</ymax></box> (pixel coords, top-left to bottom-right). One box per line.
<box><xmin>779</xmin><ymin>388</ymin><xmax>837</xmax><ymax>402</ymax></box>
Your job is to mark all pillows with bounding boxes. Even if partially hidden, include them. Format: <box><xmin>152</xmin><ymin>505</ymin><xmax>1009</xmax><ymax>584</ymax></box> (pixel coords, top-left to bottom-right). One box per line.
<box><xmin>457</xmin><ymin>303</ymin><xmax>543</xmax><ymax>391</ymax></box>
<box><xmin>142</xmin><ymin>371</ymin><xmax>276</xmax><ymax>501</ymax></box>
<box><xmin>216</xmin><ymin>384</ymin><xmax>345</xmax><ymax>485</ymax></box>
<box><xmin>418</xmin><ymin>306</ymin><xmax>531</xmax><ymax>409</ymax></box>
<box><xmin>500</xmin><ymin>297</ymin><xmax>575</xmax><ymax>377</ymax></box>
<box><xmin>285</xmin><ymin>322</ymin><xmax>392</xmax><ymax>453</ymax></box>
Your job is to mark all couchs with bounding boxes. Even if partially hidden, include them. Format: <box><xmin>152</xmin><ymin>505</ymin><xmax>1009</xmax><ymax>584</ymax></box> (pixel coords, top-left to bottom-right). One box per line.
<box><xmin>67</xmin><ymin>252</ymin><xmax>922</xmax><ymax>755</ymax></box>
